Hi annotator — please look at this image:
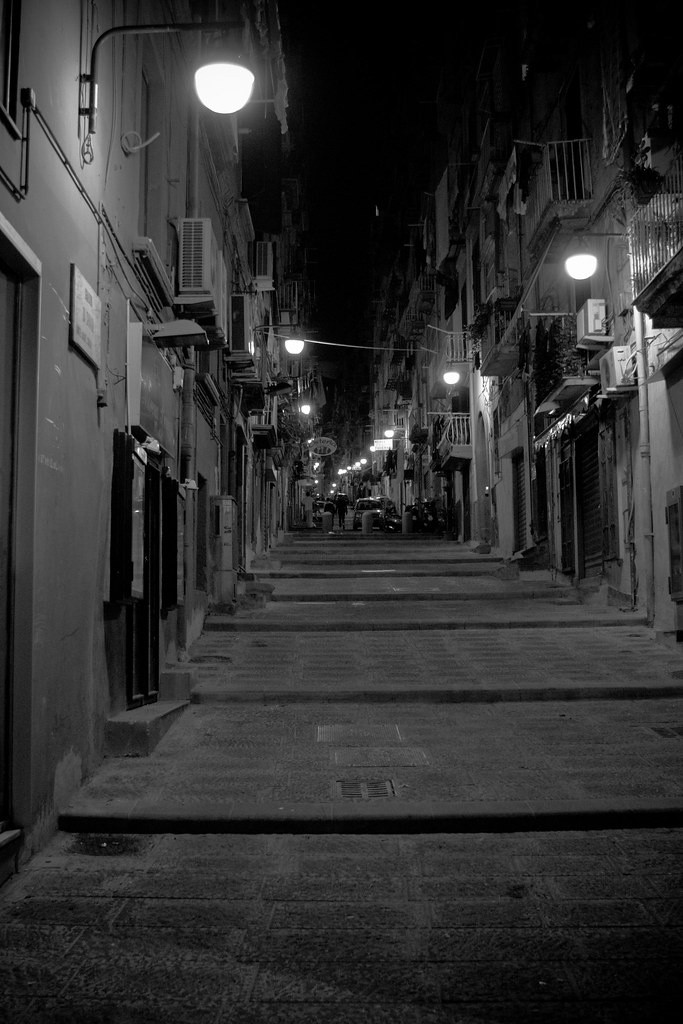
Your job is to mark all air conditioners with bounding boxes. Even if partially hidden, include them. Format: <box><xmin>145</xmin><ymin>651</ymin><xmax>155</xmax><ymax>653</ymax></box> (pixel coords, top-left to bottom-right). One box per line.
<box><xmin>255</xmin><ymin>240</ymin><xmax>275</xmax><ymax>281</ymax></box>
<box><xmin>177</xmin><ymin>216</ymin><xmax>226</xmax><ymax>343</ymax></box>
<box><xmin>599</xmin><ymin>345</ymin><xmax>635</xmax><ymax>392</ymax></box>
<box><xmin>576</xmin><ymin>297</ymin><xmax>604</xmax><ymax>341</ymax></box>
<box><xmin>230</xmin><ymin>292</ymin><xmax>255</xmax><ymax>361</ymax></box>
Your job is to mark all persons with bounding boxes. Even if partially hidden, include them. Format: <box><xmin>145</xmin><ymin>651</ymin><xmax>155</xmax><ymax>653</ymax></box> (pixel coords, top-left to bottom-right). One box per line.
<box><xmin>336</xmin><ymin>495</ymin><xmax>348</xmax><ymax>531</ymax></box>
<box><xmin>301</xmin><ymin>491</ymin><xmax>315</xmax><ymax>527</ymax></box>
<box><xmin>324</xmin><ymin>497</ymin><xmax>336</xmax><ymax>530</ymax></box>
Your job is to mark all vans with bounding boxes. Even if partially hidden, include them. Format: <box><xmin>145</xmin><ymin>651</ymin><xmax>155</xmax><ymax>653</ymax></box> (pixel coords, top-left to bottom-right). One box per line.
<box><xmin>334</xmin><ymin>493</ymin><xmax>349</xmax><ymax>505</ymax></box>
<box><xmin>351</xmin><ymin>496</ymin><xmax>397</xmax><ymax>532</ymax></box>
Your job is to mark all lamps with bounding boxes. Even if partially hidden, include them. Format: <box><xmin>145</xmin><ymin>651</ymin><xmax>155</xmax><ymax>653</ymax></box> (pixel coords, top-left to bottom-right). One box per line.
<box><xmin>266</xmin><ymin>381</ymin><xmax>291</xmax><ymax>395</ymax></box>
<box><xmin>443</xmin><ymin>359</ymin><xmax>469</xmax><ymax>384</ymax></box>
<box><xmin>78</xmin><ymin>21</ymin><xmax>255</xmax><ymax>131</ymax></box>
<box><xmin>254</xmin><ymin>324</ymin><xmax>303</xmax><ymax>354</ymax></box>
<box><xmin>563</xmin><ymin>232</ymin><xmax>624</xmax><ymax>281</ymax></box>
